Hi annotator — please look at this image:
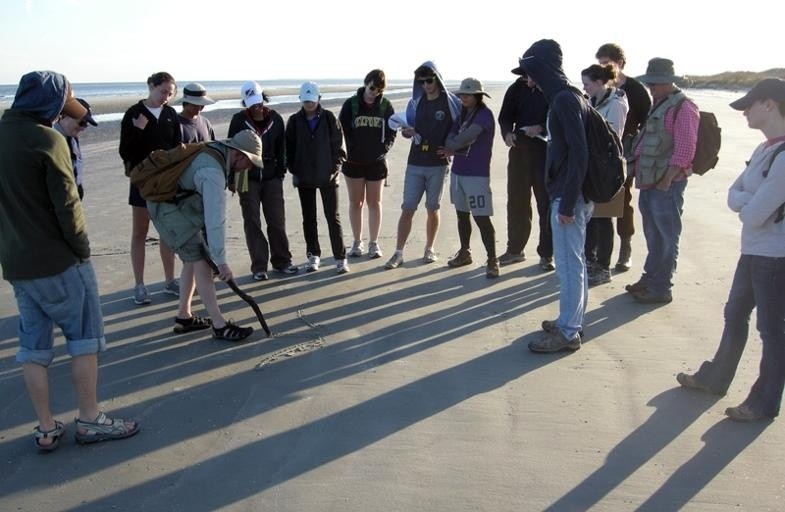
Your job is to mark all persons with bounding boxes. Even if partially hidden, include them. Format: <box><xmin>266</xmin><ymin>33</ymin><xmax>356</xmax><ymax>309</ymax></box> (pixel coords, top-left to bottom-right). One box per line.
<box><xmin>338</xmin><ymin>68</ymin><xmax>396</xmax><ymax>257</ymax></box>
<box><xmin>677</xmin><ymin>78</ymin><xmax>785</xmax><ymax>422</ymax></box>
<box><xmin>227</xmin><ymin>78</ymin><xmax>297</xmax><ymax>280</ymax></box>
<box><xmin>499</xmin><ymin>66</ymin><xmax>554</xmax><ymax>270</ymax></box>
<box><xmin>174</xmin><ymin>84</ymin><xmax>220</xmax><ymax>144</ymax></box>
<box><xmin>118</xmin><ymin>71</ymin><xmax>191</xmax><ymax>307</ymax></box>
<box><xmin>51</xmin><ymin>98</ymin><xmax>95</xmax><ymax>206</ymax></box>
<box><xmin>628</xmin><ymin>57</ymin><xmax>700</xmax><ymax>304</ymax></box>
<box><xmin>0</xmin><ymin>71</ymin><xmax>140</xmax><ymax>451</ymax></box>
<box><xmin>384</xmin><ymin>62</ymin><xmax>460</xmax><ymax>268</ymax></box>
<box><xmin>285</xmin><ymin>83</ymin><xmax>349</xmax><ymax>275</ymax></box>
<box><xmin>576</xmin><ymin>63</ymin><xmax>629</xmax><ymax>287</ymax></box>
<box><xmin>513</xmin><ymin>38</ymin><xmax>592</xmax><ymax>353</ymax></box>
<box><xmin>130</xmin><ymin>129</ymin><xmax>261</xmax><ymax>342</ymax></box>
<box><xmin>597</xmin><ymin>43</ymin><xmax>652</xmax><ymax>275</ymax></box>
<box><xmin>446</xmin><ymin>77</ymin><xmax>499</xmax><ymax>279</ymax></box>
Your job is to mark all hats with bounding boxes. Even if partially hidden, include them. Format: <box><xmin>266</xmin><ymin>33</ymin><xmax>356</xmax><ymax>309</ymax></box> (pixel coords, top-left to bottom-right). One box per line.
<box><xmin>241</xmin><ymin>80</ymin><xmax>264</xmax><ymax>109</ymax></box>
<box><xmin>214</xmin><ymin>130</ymin><xmax>264</xmax><ymax>169</ymax></box>
<box><xmin>174</xmin><ymin>83</ymin><xmax>217</xmax><ymax>106</ymax></box>
<box><xmin>730</xmin><ymin>77</ymin><xmax>783</xmax><ymax>110</ymax></box>
<box><xmin>299</xmin><ymin>81</ymin><xmax>321</xmax><ymax>102</ymax></box>
<box><xmin>62</xmin><ymin>79</ymin><xmax>98</xmax><ymax>126</ymax></box>
<box><xmin>454</xmin><ymin>77</ymin><xmax>491</xmax><ymax>99</ymax></box>
<box><xmin>635</xmin><ymin>58</ymin><xmax>683</xmax><ymax>85</ymax></box>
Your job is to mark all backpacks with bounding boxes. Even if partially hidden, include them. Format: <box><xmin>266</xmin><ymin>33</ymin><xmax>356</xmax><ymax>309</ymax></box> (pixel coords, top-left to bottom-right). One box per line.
<box><xmin>674</xmin><ymin>98</ymin><xmax>721</xmax><ymax>175</ymax></box>
<box><xmin>547</xmin><ymin>84</ymin><xmax>627</xmax><ymax>203</ymax></box>
<box><xmin>130</xmin><ymin>141</ymin><xmax>226</xmax><ymax>207</ymax></box>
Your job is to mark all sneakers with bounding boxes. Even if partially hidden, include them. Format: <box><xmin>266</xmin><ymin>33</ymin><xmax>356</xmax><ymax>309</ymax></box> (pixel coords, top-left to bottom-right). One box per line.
<box><xmin>543</xmin><ymin>320</ymin><xmax>584</xmax><ymax>339</ymax></box>
<box><xmin>724</xmin><ymin>401</ymin><xmax>776</xmax><ymax>420</ymax></box>
<box><xmin>529</xmin><ymin>332</ymin><xmax>581</xmax><ymax>353</ymax></box>
<box><xmin>305</xmin><ymin>241</ymin><xmax>382</xmax><ymax>272</ymax></box>
<box><xmin>677</xmin><ymin>372</ymin><xmax>716</xmax><ymax>392</ymax></box>
<box><xmin>271</xmin><ymin>263</ymin><xmax>299</xmax><ymax>274</ymax></box>
<box><xmin>164</xmin><ymin>280</ymin><xmax>180</xmax><ymax>297</ymax></box>
<box><xmin>386</xmin><ymin>249</ymin><xmax>499</xmax><ymax>277</ymax></box>
<box><xmin>252</xmin><ymin>272</ymin><xmax>268</xmax><ymax>280</ymax></box>
<box><xmin>134</xmin><ymin>283</ymin><xmax>151</xmax><ymax>304</ymax></box>
<box><xmin>498</xmin><ymin>244</ymin><xmax>673</xmax><ymax>303</ymax></box>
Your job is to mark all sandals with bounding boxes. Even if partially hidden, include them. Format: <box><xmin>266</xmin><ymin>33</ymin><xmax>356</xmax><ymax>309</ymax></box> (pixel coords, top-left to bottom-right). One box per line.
<box><xmin>173</xmin><ymin>311</ymin><xmax>211</xmax><ymax>334</ymax></box>
<box><xmin>33</xmin><ymin>421</ymin><xmax>66</xmax><ymax>452</ymax></box>
<box><xmin>211</xmin><ymin>319</ymin><xmax>253</xmax><ymax>341</ymax></box>
<box><xmin>74</xmin><ymin>412</ymin><xmax>140</xmax><ymax>443</ymax></box>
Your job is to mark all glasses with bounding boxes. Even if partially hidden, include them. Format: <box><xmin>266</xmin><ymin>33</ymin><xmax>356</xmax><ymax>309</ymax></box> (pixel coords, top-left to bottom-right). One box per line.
<box><xmin>368</xmin><ymin>82</ymin><xmax>384</xmax><ymax>93</ymax></box>
<box><xmin>417</xmin><ymin>77</ymin><xmax>436</xmax><ymax>85</ymax></box>
